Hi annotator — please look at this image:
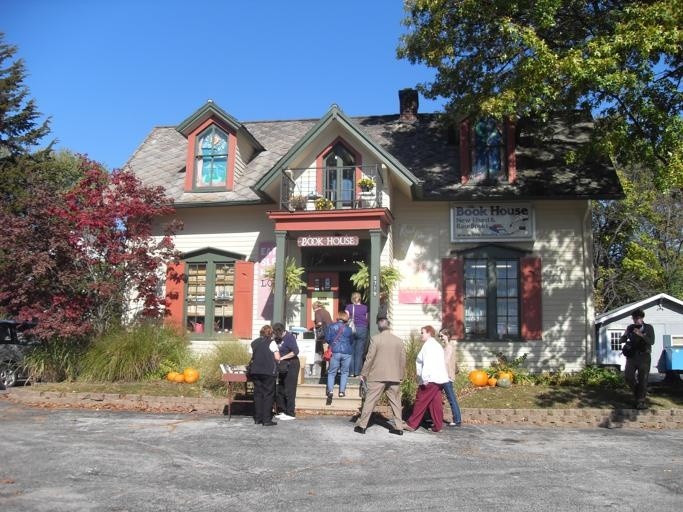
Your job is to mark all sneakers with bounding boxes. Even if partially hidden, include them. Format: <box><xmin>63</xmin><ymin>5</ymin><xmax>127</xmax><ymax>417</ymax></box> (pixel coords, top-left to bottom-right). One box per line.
<box><xmin>326</xmin><ymin>390</ymin><xmax>334</xmax><ymax>405</ymax></box>
<box><xmin>279</xmin><ymin>414</ymin><xmax>296</xmax><ymax>421</ymax></box>
<box><xmin>274</xmin><ymin>412</ymin><xmax>285</xmax><ymax>419</ymax></box>
<box><xmin>338</xmin><ymin>392</ymin><xmax>345</xmax><ymax>397</ymax></box>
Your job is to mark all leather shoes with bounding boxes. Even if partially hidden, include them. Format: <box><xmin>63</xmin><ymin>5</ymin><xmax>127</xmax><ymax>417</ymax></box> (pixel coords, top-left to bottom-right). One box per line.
<box><xmin>262</xmin><ymin>420</ymin><xmax>277</xmax><ymax>426</ymax></box>
<box><xmin>402</xmin><ymin>423</ymin><xmax>414</xmax><ymax>433</ymax></box>
<box><xmin>447</xmin><ymin>422</ymin><xmax>460</xmax><ymax>426</ymax></box>
<box><xmin>254</xmin><ymin>420</ymin><xmax>263</xmax><ymax>424</ymax></box>
<box><xmin>354</xmin><ymin>425</ymin><xmax>366</xmax><ymax>434</ymax></box>
<box><xmin>389</xmin><ymin>428</ymin><xmax>403</xmax><ymax>435</ymax></box>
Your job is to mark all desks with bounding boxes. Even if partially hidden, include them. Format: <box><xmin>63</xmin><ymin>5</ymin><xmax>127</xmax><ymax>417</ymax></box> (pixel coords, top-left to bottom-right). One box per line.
<box><xmin>221</xmin><ymin>368</ymin><xmax>280</xmax><ymax>423</ymax></box>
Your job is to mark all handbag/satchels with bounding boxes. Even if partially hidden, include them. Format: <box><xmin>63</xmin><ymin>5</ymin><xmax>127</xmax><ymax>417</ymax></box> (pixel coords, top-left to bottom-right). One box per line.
<box><xmin>278</xmin><ymin>360</ymin><xmax>290</xmax><ymax>373</ymax></box>
<box><xmin>316</xmin><ymin>321</ymin><xmax>328</xmax><ymax>340</ymax></box>
<box><xmin>348</xmin><ymin>318</ymin><xmax>357</xmax><ymax>334</ymax></box>
<box><xmin>321</xmin><ymin>347</ymin><xmax>333</xmax><ymax>361</ymax></box>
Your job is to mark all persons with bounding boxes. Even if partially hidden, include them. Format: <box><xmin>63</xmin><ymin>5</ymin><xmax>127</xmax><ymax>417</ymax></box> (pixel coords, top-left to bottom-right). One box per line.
<box><xmin>402</xmin><ymin>326</ymin><xmax>450</xmax><ymax>433</ymax></box>
<box><xmin>323</xmin><ymin>311</ymin><xmax>353</xmax><ymax>406</ymax></box>
<box><xmin>354</xmin><ymin>318</ymin><xmax>406</xmax><ymax>435</ymax></box>
<box><xmin>273</xmin><ymin>322</ymin><xmax>300</xmax><ymax>421</ymax></box>
<box><xmin>379</xmin><ymin>288</ymin><xmax>388</xmax><ymax>319</ymax></box>
<box><xmin>312</xmin><ymin>301</ymin><xmax>333</xmax><ymax>377</ymax></box>
<box><xmin>437</xmin><ymin>327</ymin><xmax>462</xmax><ymax>426</ymax></box>
<box><xmin>345</xmin><ymin>291</ymin><xmax>369</xmax><ymax>376</ymax></box>
<box><xmin>250</xmin><ymin>325</ymin><xmax>281</xmax><ymax>425</ymax></box>
<box><xmin>619</xmin><ymin>309</ymin><xmax>655</xmax><ymax>409</ymax></box>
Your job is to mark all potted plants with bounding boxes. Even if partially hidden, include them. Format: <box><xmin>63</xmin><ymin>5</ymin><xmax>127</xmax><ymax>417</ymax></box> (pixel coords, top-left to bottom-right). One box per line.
<box><xmin>356</xmin><ymin>176</ymin><xmax>376</xmax><ymax>211</ymax></box>
<box><xmin>287</xmin><ymin>191</ymin><xmax>335</xmax><ymax>212</ymax></box>
